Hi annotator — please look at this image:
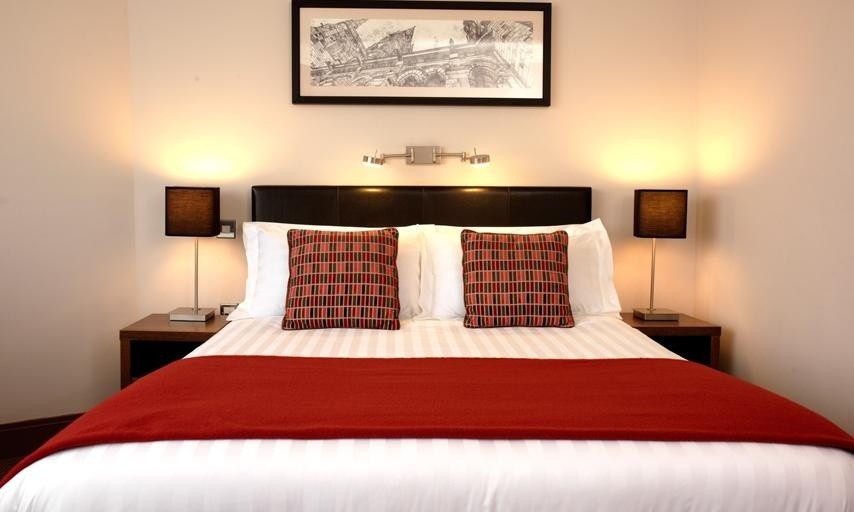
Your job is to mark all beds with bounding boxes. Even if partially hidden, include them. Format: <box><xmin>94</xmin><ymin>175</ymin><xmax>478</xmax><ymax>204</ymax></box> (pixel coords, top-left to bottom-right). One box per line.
<box><xmin>1</xmin><ymin>189</ymin><xmax>853</xmax><ymax>510</ymax></box>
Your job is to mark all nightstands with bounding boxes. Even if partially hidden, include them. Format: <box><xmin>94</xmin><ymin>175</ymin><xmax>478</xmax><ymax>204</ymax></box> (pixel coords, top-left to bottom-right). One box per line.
<box><xmin>620</xmin><ymin>311</ymin><xmax>723</xmax><ymax>370</ymax></box>
<box><xmin>118</xmin><ymin>313</ymin><xmax>233</xmax><ymax>390</ymax></box>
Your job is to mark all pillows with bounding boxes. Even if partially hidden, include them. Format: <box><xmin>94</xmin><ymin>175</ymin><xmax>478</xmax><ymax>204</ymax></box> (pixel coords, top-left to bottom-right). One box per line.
<box><xmin>280</xmin><ymin>225</ymin><xmax>402</xmax><ymax>330</ymax></box>
<box><xmin>422</xmin><ymin>221</ymin><xmax>622</xmax><ymax>330</ymax></box>
<box><xmin>226</xmin><ymin>221</ymin><xmax>421</xmax><ymax>328</ymax></box>
<box><xmin>459</xmin><ymin>228</ymin><xmax>576</xmax><ymax>330</ymax></box>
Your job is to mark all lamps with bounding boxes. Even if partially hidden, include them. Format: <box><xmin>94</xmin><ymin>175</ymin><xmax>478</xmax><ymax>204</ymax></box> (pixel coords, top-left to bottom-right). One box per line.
<box><xmin>632</xmin><ymin>189</ymin><xmax>688</xmax><ymax>322</ymax></box>
<box><xmin>164</xmin><ymin>186</ymin><xmax>222</xmax><ymax>323</ymax></box>
<box><xmin>361</xmin><ymin>145</ymin><xmax>492</xmax><ymax>170</ymax></box>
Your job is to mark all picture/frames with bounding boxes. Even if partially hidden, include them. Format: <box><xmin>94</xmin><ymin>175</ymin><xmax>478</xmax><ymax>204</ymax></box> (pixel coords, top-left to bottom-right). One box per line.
<box><xmin>290</xmin><ymin>3</ymin><xmax>552</xmax><ymax>107</ymax></box>
